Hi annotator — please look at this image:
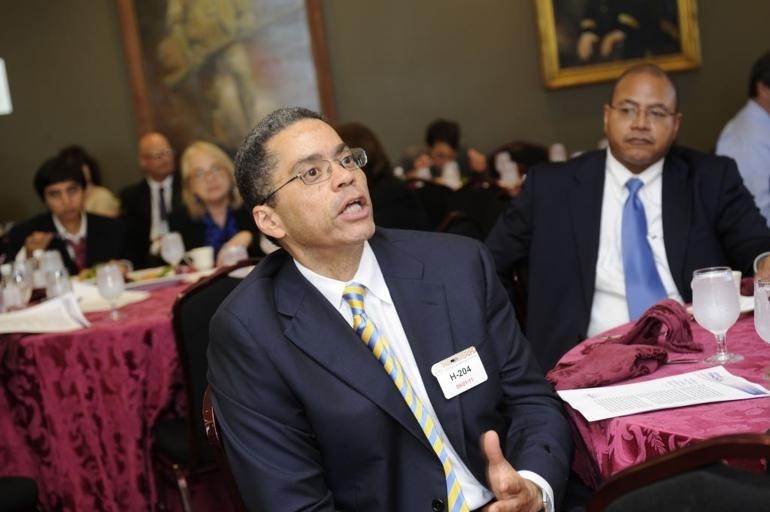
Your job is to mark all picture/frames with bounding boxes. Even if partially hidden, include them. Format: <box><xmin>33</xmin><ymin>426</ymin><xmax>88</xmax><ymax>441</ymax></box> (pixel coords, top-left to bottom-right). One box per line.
<box><xmin>120</xmin><ymin>1</ymin><xmax>341</xmax><ymax>167</ymax></box>
<box><xmin>536</xmin><ymin>0</ymin><xmax>704</xmax><ymax>92</ymax></box>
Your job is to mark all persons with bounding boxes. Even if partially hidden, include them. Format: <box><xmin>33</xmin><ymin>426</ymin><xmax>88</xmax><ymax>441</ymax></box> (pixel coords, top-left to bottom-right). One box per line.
<box><xmin>333</xmin><ymin>121</ymin><xmax>403</xmax><ymax>210</ymax></box>
<box><xmin>62</xmin><ymin>147</ymin><xmax>119</xmax><ymax>218</ymax></box>
<box><xmin>209</xmin><ymin>104</ymin><xmax>573</xmax><ymax>512</ymax></box>
<box><xmin>716</xmin><ymin>53</ymin><xmax>769</xmax><ymax>220</ymax></box>
<box><xmin>116</xmin><ymin>132</ymin><xmax>187</xmax><ymax>266</ymax></box>
<box><xmin>410</xmin><ymin>117</ymin><xmax>475</xmax><ymax>192</ymax></box>
<box><xmin>2</xmin><ymin>160</ymin><xmax>119</xmax><ymax>275</ymax></box>
<box><xmin>488</xmin><ymin>63</ymin><xmax>769</xmax><ymax>368</ymax></box>
<box><xmin>173</xmin><ymin>142</ymin><xmax>250</xmax><ymax>258</ymax></box>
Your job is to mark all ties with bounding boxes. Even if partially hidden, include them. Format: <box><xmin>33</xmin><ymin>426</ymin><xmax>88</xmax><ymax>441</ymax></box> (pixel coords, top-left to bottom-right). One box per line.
<box><xmin>343</xmin><ymin>284</ymin><xmax>469</xmax><ymax>512</ymax></box>
<box><xmin>68</xmin><ymin>237</ymin><xmax>87</xmax><ymax>269</ymax></box>
<box><xmin>620</xmin><ymin>179</ymin><xmax>668</xmax><ymax>321</ymax></box>
<box><xmin>159</xmin><ymin>187</ymin><xmax>168</xmax><ymax>222</ymax></box>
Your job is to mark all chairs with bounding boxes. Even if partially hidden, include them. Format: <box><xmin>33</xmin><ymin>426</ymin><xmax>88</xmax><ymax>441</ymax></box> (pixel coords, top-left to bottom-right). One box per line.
<box><xmin>151</xmin><ymin>256</ymin><xmax>262</xmax><ymax>511</ymax></box>
<box><xmin>198</xmin><ymin>378</ymin><xmax>260</xmax><ymax>511</ymax></box>
<box><xmin>506</xmin><ymin>263</ymin><xmax>531</xmax><ymax>336</ymax></box>
<box><xmin>429</xmin><ymin>210</ymin><xmax>478</xmax><ymax>232</ymax></box>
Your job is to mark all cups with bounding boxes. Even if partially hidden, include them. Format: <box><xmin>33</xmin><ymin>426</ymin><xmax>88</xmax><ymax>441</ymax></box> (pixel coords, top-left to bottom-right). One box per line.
<box><xmin>691</xmin><ymin>268</ymin><xmax>743</xmax><ymax>363</ymax></box>
<box><xmin>161</xmin><ymin>233</ymin><xmax>186</xmax><ymax>268</ymax></box>
<box><xmin>95</xmin><ymin>266</ymin><xmax>129</xmax><ymax>321</ymax></box>
<box><xmin>46</xmin><ymin>268</ymin><xmax>69</xmax><ymax>298</ymax></box>
<box><xmin>10</xmin><ymin>272</ymin><xmax>32</xmax><ymax>305</ymax></box>
<box><xmin>183</xmin><ymin>246</ymin><xmax>215</xmax><ymax>272</ymax></box>
<box><xmin>753</xmin><ymin>252</ymin><xmax>770</xmax><ymax>380</ymax></box>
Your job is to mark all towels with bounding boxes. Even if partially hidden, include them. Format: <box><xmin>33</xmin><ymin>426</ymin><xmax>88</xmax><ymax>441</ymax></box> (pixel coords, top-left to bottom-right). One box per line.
<box><xmin>544</xmin><ymin>339</ymin><xmax>670</xmax><ymax>389</ymax></box>
<box><xmin>609</xmin><ymin>293</ymin><xmax>704</xmax><ymax>355</ymax></box>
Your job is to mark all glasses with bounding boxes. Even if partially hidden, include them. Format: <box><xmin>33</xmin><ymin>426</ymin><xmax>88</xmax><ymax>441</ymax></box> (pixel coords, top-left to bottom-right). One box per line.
<box><xmin>259</xmin><ymin>147</ymin><xmax>368</xmax><ymax>201</ymax></box>
<box><xmin>610</xmin><ymin>104</ymin><xmax>676</xmax><ymax>123</ymax></box>
